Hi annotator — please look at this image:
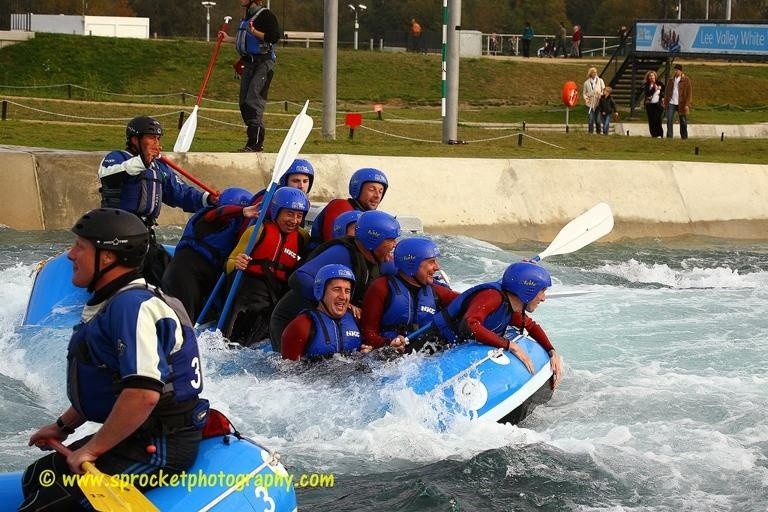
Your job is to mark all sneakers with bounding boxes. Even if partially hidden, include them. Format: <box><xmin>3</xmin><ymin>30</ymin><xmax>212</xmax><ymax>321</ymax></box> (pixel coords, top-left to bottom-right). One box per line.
<box><xmin>238</xmin><ymin>143</ymin><xmax>262</xmax><ymax>152</ymax></box>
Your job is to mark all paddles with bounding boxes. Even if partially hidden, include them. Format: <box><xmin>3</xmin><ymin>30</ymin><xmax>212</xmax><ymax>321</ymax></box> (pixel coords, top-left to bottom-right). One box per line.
<box><xmin>213</xmin><ymin>99</ymin><xmax>310</xmax><ymax>341</ymax></box>
<box><xmin>172</xmin><ymin>15</ymin><xmax>233</xmax><ymax>153</ymax></box>
<box><xmin>44</xmin><ymin>439</ymin><xmax>161</xmax><ymax>512</ymax></box>
<box><xmin>195</xmin><ymin>114</ymin><xmax>312</xmax><ymax>327</ymax></box>
<box><xmin>394</xmin><ymin>201</ymin><xmax>614</xmax><ymax>348</ymax></box>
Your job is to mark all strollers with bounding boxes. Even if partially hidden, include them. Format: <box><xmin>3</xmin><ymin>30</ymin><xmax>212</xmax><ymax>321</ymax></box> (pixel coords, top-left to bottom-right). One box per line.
<box><xmin>535</xmin><ymin>34</ymin><xmax>557</xmax><ymax>58</ymax></box>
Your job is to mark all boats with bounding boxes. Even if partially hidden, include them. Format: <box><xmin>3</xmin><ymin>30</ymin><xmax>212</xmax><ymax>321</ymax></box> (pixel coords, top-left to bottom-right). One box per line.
<box><xmin>2</xmin><ymin>426</ymin><xmax>299</xmax><ymax>512</ymax></box>
<box><xmin>26</xmin><ymin>232</ymin><xmax>556</xmax><ymax>468</ymax></box>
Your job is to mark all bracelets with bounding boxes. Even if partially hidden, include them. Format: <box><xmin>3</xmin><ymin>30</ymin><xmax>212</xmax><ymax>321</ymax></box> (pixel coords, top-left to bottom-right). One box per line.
<box><xmin>56</xmin><ymin>416</ymin><xmax>75</xmax><ymax>434</ymax></box>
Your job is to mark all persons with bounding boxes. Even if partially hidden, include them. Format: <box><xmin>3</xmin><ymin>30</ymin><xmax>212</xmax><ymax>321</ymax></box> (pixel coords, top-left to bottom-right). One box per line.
<box><xmin>158</xmin><ymin>183</ymin><xmax>262</xmax><ymax>319</ymax></box>
<box><xmin>409</xmin><ymin>18</ymin><xmax>423</xmax><ymax>53</ymax></box>
<box><xmin>226</xmin><ymin>154</ymin><xmax>462</xmax><ymax>372</ymax></box>
<box><xmin>486</xmin><ymin>19</ymin><xmax>584</xmax><ymax>60</ymax></box>
<box><xmin>217</xmin><ymin>0</ymin><xmax>281</xmax><ymax>152</ymax></box>
<box><xmin>96</xmin><ymin>115</ymin><xmax>218</xmax><ymax>290</ymax></box>
<box><xmin>644</xmin><ymin>69</ymin><xmax>665</xmax><ymax>139</ymax></box>
<box><xmin>412</xmin><ymin>261</ymin><xmax>563</xmax><ymax>383</ymax></box>
<box><xmin>582</xmin><ymin>67</ymin><xmax>605</xmax><ymax>135</ymax></box>
<box><xmin>14</xmin><ymin>205</ymin><xmax>205</xmax><ymax>510</ymax></box>
<box><xmin>662</xmin><ymin>63</ymin><xmax>693</xmax><ymax>139</ymax></box>
<box><xmin>598</xmin><ymin>87</ymin><xmax>618</xmax><ymax>135</ymax></box>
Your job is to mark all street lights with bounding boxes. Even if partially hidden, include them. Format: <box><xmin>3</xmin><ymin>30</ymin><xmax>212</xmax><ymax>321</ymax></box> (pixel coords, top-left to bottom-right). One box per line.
<box><xmin>347</xmin><ymin>2</ymin><xmax>368</xmax><ymax>51</ymax></box>
<box><xmin>201</xmin><ymin>0</ymin><xmax>216</xmax><ymax>42</ymax></box>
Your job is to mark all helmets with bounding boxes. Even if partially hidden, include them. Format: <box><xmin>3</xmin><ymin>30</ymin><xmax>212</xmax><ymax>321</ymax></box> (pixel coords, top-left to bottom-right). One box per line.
<box><xmin>394</xmin><ymin>237</ymin><xmax>440</xmax><ymax>276</ymax></box>
<box><xmin>218</xmin><ymin>188</ymin><xmax>252</xmax><ymax>206</ymax></box>
<box><xmin>271</xmin><ymin>159</ymin><xmax>314</xmax><ymax>224</ymax></box>
<box><xmin>502</xmin><ymin>263</ymin><xmax>552</xmax><ymax>304</ymax></box>
<box><xmin>349</xmin><ymin>168</ymin><xmax>388</xmax><ymax>199</ymax></box>
<box><xmin>72</xmin><ymin>208</ymin><xmax>150</xmax><ymax>266</ymax></box>
<box><xmin>126</xmin><ymin>116</ymin><xmax>162</xmax><ymax>138</ymax></box>
<box><xmin>313</xmin><ymin>263</ymin><xmax>356</xmax><ymax>301</ymax></box>
<box><xmin>332</xmin><ymin>210</ymin><xmax>401</xmax><ymax>251</ymax></box>
